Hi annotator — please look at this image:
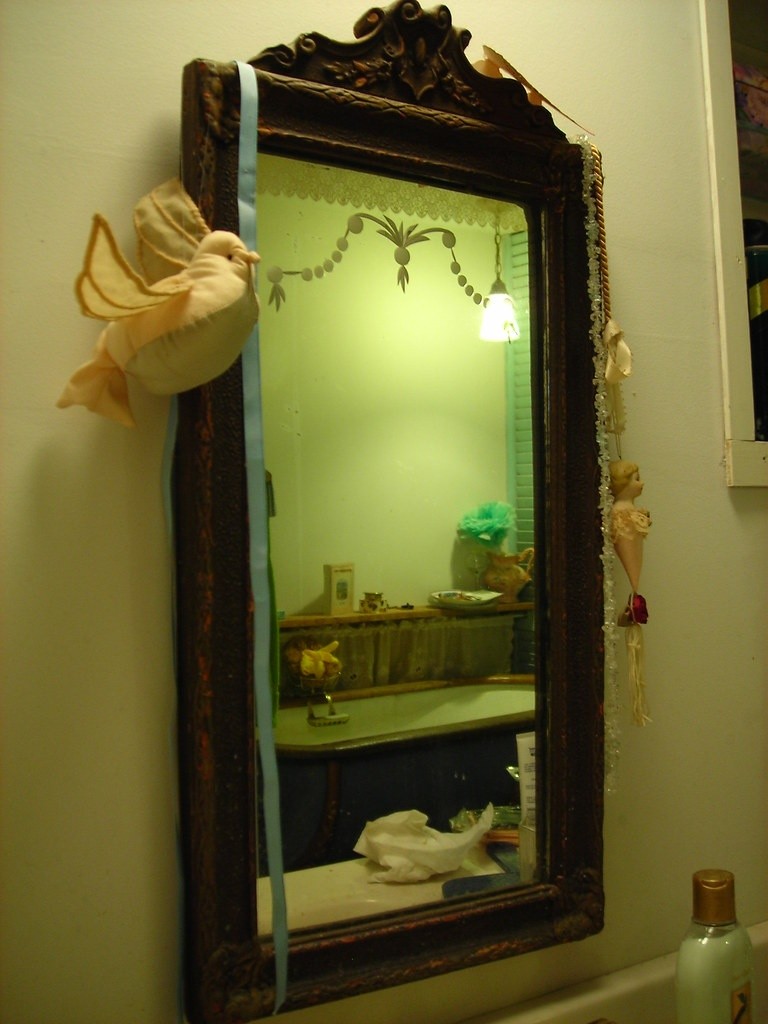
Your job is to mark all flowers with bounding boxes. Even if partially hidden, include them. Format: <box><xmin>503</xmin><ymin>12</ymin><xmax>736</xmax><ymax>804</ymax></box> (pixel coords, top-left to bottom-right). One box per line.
<box><xmin>458</xmin><ymin>501</ymin><xmax>517</xmax><ymax>549</ymax></box>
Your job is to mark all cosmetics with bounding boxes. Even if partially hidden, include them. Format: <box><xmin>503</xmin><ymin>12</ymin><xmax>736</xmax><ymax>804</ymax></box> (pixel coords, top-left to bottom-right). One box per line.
<box><xmin>674</xmin><ymin>868</ymin><xmax>760</xmax><ymax>1024</ymax></box>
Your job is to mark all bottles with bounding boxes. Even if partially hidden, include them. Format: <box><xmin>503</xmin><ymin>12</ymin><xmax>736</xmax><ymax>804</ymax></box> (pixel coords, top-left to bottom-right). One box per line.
<box><xmin>676</xmin><ymin>869</ymin><xmax>757</xmax><ymax>1024</ymax></box>
<box><xmin>517</xmin><ymin>805</ymin><xmax>537</xmax><ymax>881</ymax></box>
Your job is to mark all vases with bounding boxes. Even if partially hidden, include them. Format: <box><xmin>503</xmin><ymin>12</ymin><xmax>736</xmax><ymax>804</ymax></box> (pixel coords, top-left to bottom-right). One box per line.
<box><xmin>470</xmin><ymin>549</ymin><xmax>499</xmax><ymax>589</ymax></box>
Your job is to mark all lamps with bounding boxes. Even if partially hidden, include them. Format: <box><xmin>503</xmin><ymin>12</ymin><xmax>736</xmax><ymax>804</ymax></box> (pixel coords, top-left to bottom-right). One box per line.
<box><xmin>480</xmin><ymin>223</ymin><xmax>520</xmax><ymax>343</ymax></box>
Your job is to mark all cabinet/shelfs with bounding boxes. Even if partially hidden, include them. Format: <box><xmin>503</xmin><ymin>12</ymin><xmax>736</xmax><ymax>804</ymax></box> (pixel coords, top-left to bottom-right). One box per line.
<box><xmin>279</xmin><ymin>602</ymin><xmax>536</xmax><ymax>709</ymax></box>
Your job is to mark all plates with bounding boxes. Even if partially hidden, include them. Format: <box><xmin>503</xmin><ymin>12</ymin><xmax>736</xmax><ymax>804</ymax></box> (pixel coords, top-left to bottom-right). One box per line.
<box><xmin>429</xmin><ymin>589</ymin><xmax>505</xmax><ymax>611</ymax></box>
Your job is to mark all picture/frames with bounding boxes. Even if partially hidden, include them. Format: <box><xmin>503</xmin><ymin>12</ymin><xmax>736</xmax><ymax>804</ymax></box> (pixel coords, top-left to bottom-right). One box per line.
<box><xmin>699</xmin><ymin>1</ymin><xmax>768</xmax><ymax>487</ymax></box>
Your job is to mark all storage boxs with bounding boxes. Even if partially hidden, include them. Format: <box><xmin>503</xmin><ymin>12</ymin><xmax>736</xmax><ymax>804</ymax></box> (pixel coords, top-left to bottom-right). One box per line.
<box><xmin>520</xmin><ymin>826</ymin><xmax>535</xmax><ymax>883</ymax></box>
<box><xmin>324</xmin><ymin>563</ymin><xmax>354</xmax><ymax>616</ymax></box>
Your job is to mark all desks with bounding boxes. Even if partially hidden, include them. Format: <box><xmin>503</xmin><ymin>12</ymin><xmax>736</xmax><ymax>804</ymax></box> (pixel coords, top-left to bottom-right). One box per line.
<box><xmin>257</xmin><ymin>843</ymin><xmax>506</xmax><ymax>935</ymax></box>
<box><xmin>254</xmin><ymin>684</ymin><xmax>536</xmax><ymax>870</ymax></box>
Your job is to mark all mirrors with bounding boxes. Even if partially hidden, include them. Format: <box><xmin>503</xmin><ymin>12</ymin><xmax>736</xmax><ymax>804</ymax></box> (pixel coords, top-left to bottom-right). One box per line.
<box><xmin>181</xmin><ymin>1</ymin><xmax>606</xmax><ymax>1024</ymax></box>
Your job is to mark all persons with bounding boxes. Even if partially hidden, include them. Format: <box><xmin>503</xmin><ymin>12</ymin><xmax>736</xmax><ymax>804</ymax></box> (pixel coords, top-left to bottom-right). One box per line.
<box><xmin>608</xmin><ymin>461</ymin><xmax>653</xmax><ymax>593</ymax></box>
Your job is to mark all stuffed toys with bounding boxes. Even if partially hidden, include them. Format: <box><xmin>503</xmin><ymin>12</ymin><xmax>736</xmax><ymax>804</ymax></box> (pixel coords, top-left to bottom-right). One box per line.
<box><xmin>54</xmin><ymin>178</ymin><xmax>261</xmax><ymax>427</ymax></box>
